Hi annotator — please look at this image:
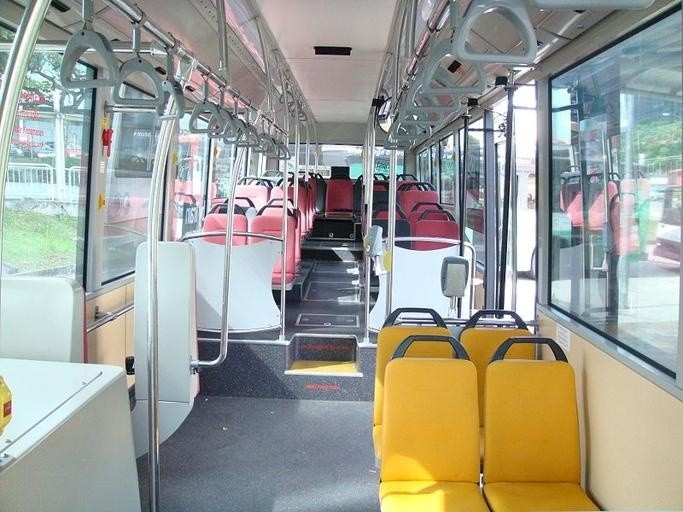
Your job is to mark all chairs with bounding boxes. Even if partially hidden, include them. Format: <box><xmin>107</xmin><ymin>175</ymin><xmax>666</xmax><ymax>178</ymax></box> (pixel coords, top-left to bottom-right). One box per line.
<box><xmin>315</xmin><ymin>173</ymin><xmax>326</xmax><ymax>213</ymax></box>
<box><xmin>372</xmin><ymin>308</ymin><xmax>455</xmax><ymax>460</ymax></box>
<box><xmin>586</xmin><ymin>175</ymin><xmax>618</xmax><ymax>230</ymax></box>
<box><xmin>354</xmin><ymin>181</ymin><xmax>401</xmax><ymax>222</ymax></box>
<box><xmin>409</xmin><ymin>202</ymin><xmax>448</xmax><ymax>224</ymax></box>
<box><xmin>223</xmin><ymin>197</ymin><xmax>255</xmax><ymax>244</ymax></box>
<box><xmin>378</xmin><ymin>334</ymin><xmax>489</xmax><ymax>512</ymax></box>
<box><xmin>262</xmin><ymin>198</ymin><xmax>301</xmax><ymax>275</ymax></box>
<box><xmin>201</xmin><ymin>204</ymin><xmax>248</xmax><ymax>246</ymax></box>
<box><xmin>359</xmin><ymin>203</ymin><xmax>411</xmax><ymax>303</ymax></box>
<box><xmin>248</xmin><ymin>205</ymin><xmax>298</xmax><ymax>291</ymax></box>
<box><xmin>412</xmin><ymin>210</ymin><xmax>460</xmax><ymax>251</ymax></box>
<box><xmin>372</xmin><ymin>202</ymin><xmax>407</xmax><ymax>219</ymax></box>
<box><xmin>234</xmin><ymin>177</ymin><xmax>269</xmax><ymax>211</ymax></box>
<box><xmin>399</xmin><ymin>182</ymin><xmax>438</xmax><ymax>215</ymax></box>
<box><xmin>560</xmin><ymin>176</ymin><xmax>585</xmax><ymax>227</ymax></box>
<box><xmin>325</xmin><ymin>173</ymin><xmax>354</xmax><ymax>219</ymax></box>
<box><xmin>361</xmin><ymin>203</ymin><xmax>402</xmax><ymax>237</ymax></box>
<box><xmin>459</xmin><ymin>309</ymin><xmax>537</xmax><ymax>474</ymax></box>
<box><xmin>270</xmin><ymin>178</ymin><xmax>308</xmax><ymax>238</ymax></box>
<box><xmin>484</xmin><ymin>338</ymin><xmax>598</xmax><ymax>511</ymax></box>
<box><xmin>354</xmin><ymin>175</ymin><xmax>378</xmax><ymax>219</ymax></box>
